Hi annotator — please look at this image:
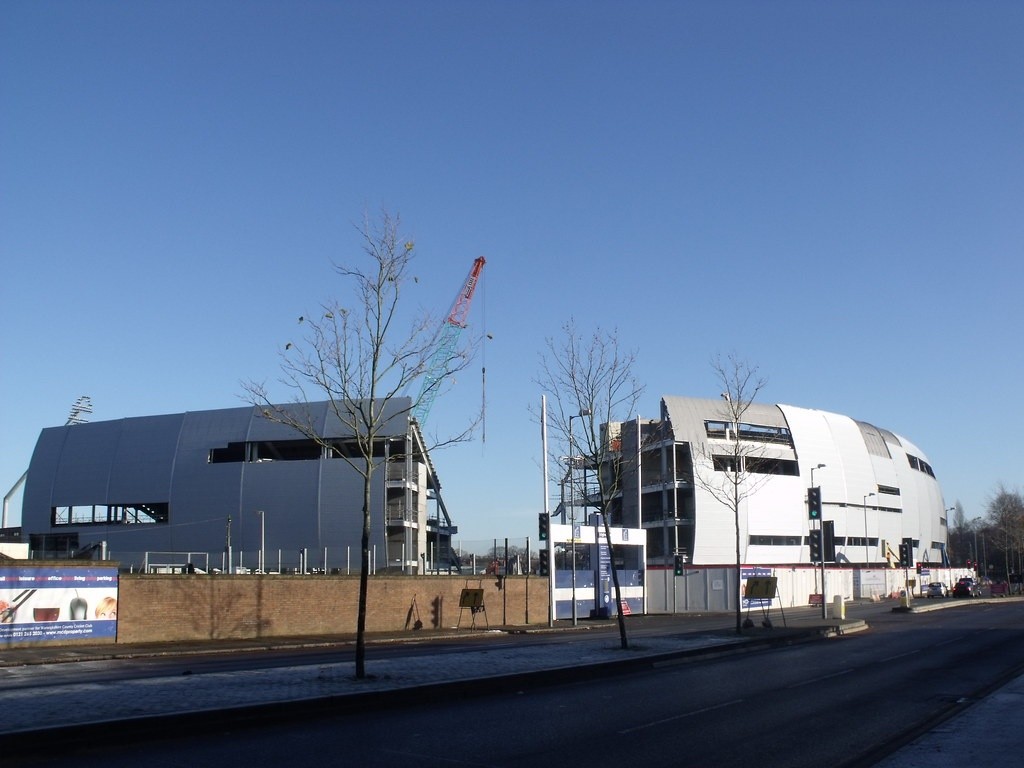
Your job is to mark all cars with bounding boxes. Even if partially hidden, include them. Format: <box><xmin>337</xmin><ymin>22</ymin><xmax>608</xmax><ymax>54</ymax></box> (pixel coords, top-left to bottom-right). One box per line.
<box><xmin>425</xmin><ymin>565</ymin><xmax>488</xmax><ymax>577</ymax></box>
<box><xmin>952</xmin><ymin>581</ymin><xmax>979</xmax><ymax>599</ymax></box>
<box><xmin>927</xmin><ymin>581</ymin><xmax>949</xmax><ymax>598</ymax></box>
<box><xmin>959</xmin><ymin>578</ymin><xmax>979</xmax><ymax>589</ymax></box>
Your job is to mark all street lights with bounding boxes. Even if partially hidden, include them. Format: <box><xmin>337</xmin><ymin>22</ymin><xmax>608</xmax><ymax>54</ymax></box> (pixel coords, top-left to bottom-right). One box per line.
<box><xmin>720</xmin><ymin>391</ymin><xmax>745</xmax><ymax>612</ymax></box>
<box><xmin>255</xmin><ymin>509</ymin><xmax>267</xmax><ymax>574</ymax></box>
<box><xmin>971</xmin><ymin>516</ymin><xmax>982</xmax><ymax>577</ymax></box>
<box><xmin>943</xmin><ymin>507</ymin><xmax>956</xmax><ymax>568</ymax></box>
<box><xmin>569</xmin><ymin>408</ymin><xmax>594</xmax><ymax>626</ymax></box>
<box><xmin>811</xmin><ymin>463</ymin><xmax>826</xmax><ymax>531</ymax></box>
<box><xmin>864</xmin><ymin>492</ymin><xmax>876</xmax><ymax>568</ymax></box>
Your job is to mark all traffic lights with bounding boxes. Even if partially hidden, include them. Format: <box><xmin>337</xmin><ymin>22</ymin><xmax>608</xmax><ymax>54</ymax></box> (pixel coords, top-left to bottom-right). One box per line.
<box><xmin>899</xmin><ymin>545</ymin><xmax>910</xmax><ymax>569</ymax></box>
<box><xmin>967</xmin><ymin>559</ymin><xmax>971</xmax><ymax>569</ymax></box>
<box><xmin>807</xmin><ymin>488</ymin><xmax>821</xmax><ymax>520</ymax></box>
<box><xmin>916</xmin><ymin>563</ymin><xmax>922</xmax><ymax>574</ymax></box>
<box><xmin>539</xmin><ymin>549</ymin><xmax>550</xmax><ymax>578</ymax></box>
<box><xmin>539</xmin><ymin>512</ymin><xmax>550</xmax><ymax>540</ymax></box>
<box><xmin>675</xmin><ymin>554</ymin><xmax>683</xmax><ymax>576</ymax></box>
<box><xmin>638</xmin><ymin>570</ymin><xmax>644</xmax><ymax>587</ymax></box>
<box><xmin>810</xmin><ymin>529</ymin><xmax>822</xmax><ymax>562</ymax></box>
<box><xmin>974</xmin><ymin>562</ymin><xmax>978</xmax><ymax>571</ymax></box>
